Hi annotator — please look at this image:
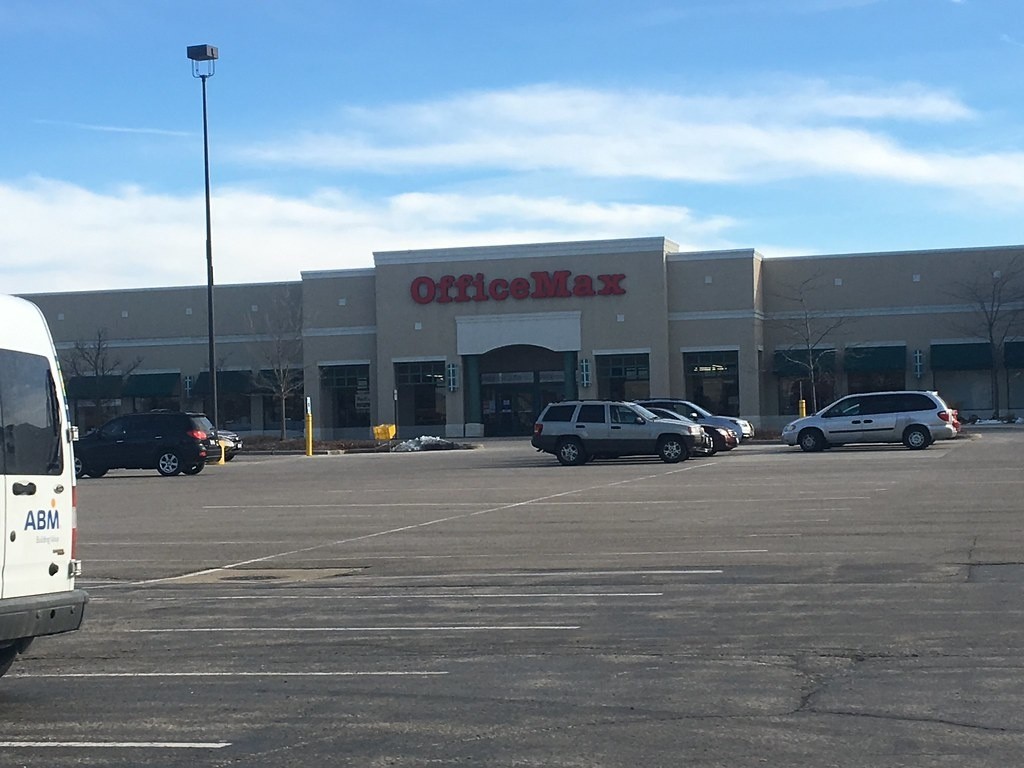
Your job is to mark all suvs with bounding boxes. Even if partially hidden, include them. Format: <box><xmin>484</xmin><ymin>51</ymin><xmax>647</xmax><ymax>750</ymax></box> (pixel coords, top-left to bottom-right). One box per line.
<box><xmin>531</xmin><ymin>400</ymin><xmax>706</xmax><ymax>466</ymax></box>
<box><xmin>73</xmin><ymin>409</ymin><xmax>222</xmax><ymax>479</ymax></box>
<box><xmin>635</xmin><ymin>399</ymin><xmax>755</xmax><ymax>438</ymax></box>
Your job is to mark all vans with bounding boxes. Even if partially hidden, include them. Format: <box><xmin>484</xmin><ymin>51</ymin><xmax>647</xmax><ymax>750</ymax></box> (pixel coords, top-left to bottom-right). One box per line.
<box><xmin>780</xmin><ymin>391</ymin><xmax>957</xmax><ymax>450</ymax></box>
<box><xmin>1</xmin><ymin>293</ymin><xmax>89</xmax><ymax>682</ymax></box>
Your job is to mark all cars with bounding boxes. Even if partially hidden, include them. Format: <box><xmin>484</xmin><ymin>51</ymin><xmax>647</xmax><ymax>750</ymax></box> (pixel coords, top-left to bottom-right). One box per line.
<box><xmin>216</xmin><ymin>430</ymin><xmax>244</xmax><ymax>463</ymax></box>
<box><xmin>644</xmin><ymin>407</ymin><xmax>740</xmax><ymax>458</ymax></box>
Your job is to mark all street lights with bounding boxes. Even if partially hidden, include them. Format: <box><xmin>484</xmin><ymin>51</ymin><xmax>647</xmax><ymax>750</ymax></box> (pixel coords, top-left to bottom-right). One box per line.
<box><xmin>187</xmin><ymin>44</ymin><xmax>227</xmax><ymax>465</ymax></box>
